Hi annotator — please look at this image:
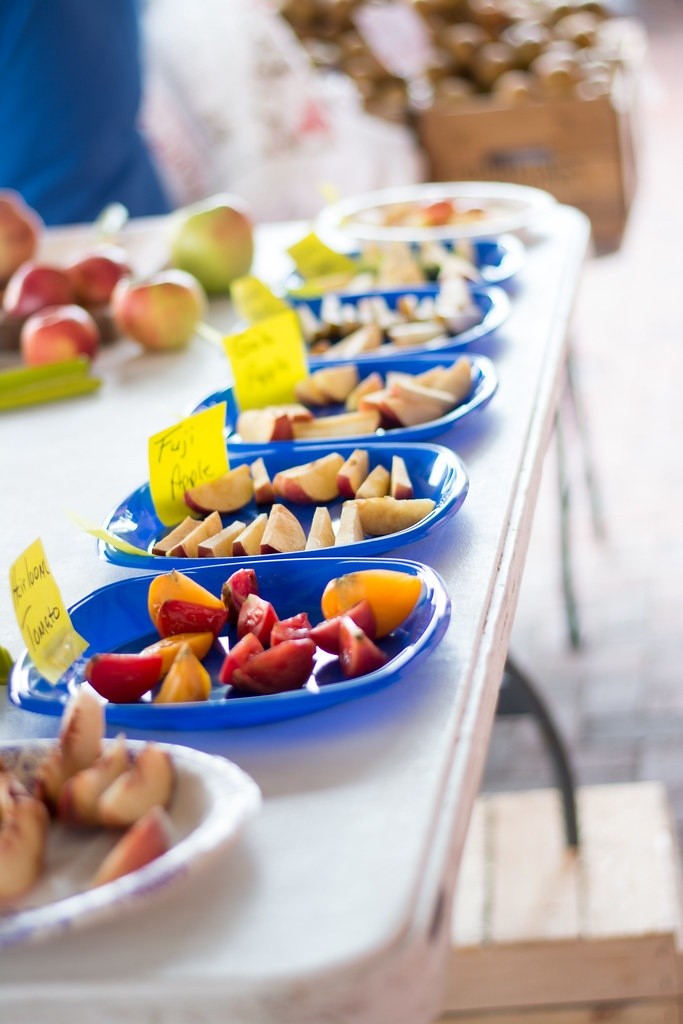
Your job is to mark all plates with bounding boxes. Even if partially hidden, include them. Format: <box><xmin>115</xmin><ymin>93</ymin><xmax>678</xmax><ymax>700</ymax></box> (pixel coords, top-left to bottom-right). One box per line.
<box><xmin>97</xmin><ymin>443</ymin><xmax>469</xmax><ymax>572</ymax></box>
<box><xmin>316</xmin><ymin>181</ymin><xmax>557</xmax><ymax>238</ymax></box>
<box><xmin>294</xmin><ymin>285</ymin><xmax>511</xmax><ymax>364</ymax></box>
<box><xmin>191</xmin><ymin>353</ymin><xmax>499</xmax><ymax>443</ymax></box>
<box><xmin>7</xmin><ymin>557</ymin><xmax>451</xmax><ymax>730</ymax></box>
<box><xmin>292</xmin><ymin>239</ymin><xmax>525</xmax><ymax>284</ymax></box>
<box><xmin>1</xmin><ymin>738</ymin><xmax>261</xmax><ymax>945</ymax></box>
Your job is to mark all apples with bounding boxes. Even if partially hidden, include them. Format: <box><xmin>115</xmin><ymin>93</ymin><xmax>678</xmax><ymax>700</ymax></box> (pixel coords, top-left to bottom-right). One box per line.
<box><xmin>237</xmin><ymin>358</ymin><xmax>476</xmax><ymax>441</ymax></box>
<box><xmin>150</xmin><ymin>447</ymin><xmax>440</xmax><ymax>558</ymax></box>
<box><xmin>0</xmin><ymin>189</ymin><xmax>253</xmax><ymax>373</ymax></box>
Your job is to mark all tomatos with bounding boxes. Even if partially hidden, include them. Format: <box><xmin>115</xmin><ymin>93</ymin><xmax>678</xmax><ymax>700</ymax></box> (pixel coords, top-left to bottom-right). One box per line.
<box><xmin>217</xmin><ymin>593</ymin><xmax>385</xmax><ymax>691</ymax></box>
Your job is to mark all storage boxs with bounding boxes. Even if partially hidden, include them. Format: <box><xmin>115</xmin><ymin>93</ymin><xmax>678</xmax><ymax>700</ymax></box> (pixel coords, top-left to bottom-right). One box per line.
<box><xmin>424</xmin><ymin>55</ymin><xmax>639</xmax><ymax>255</ymax></box>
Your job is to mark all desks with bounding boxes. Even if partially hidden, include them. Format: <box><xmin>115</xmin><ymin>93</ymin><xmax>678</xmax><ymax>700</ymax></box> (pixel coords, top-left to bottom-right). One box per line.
<box><xmin>0</xmin><ymin>201</ymin><xmax>609</xmax><ymax>1024</ymax></box>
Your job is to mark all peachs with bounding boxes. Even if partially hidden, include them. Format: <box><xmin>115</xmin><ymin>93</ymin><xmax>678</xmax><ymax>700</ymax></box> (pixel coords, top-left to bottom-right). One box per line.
<box><xmin>1</xmin><ymin>682</ymin><xmax>177</xmax><ymax>916</ymax></box>
